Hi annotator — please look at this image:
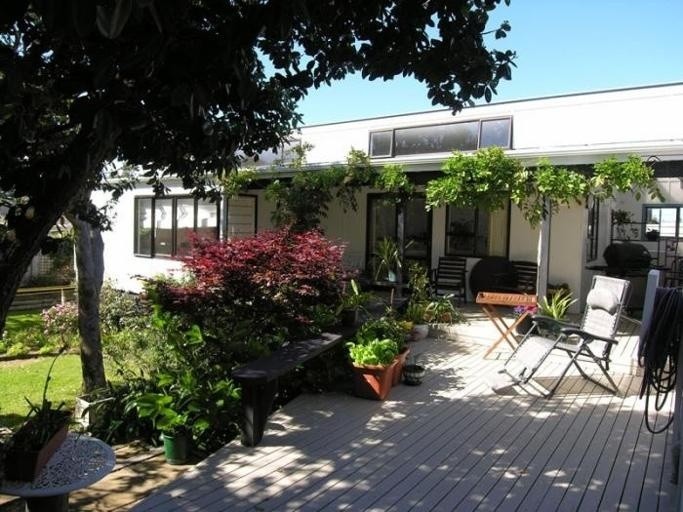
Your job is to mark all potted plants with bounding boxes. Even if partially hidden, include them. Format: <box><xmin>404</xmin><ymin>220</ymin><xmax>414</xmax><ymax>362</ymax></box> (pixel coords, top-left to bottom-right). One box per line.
<box><xmin>122</xmin><ymin>304</ymin><xmax>242</xmax><ymax>465</ymax></box>
<box><xmin>369</xmin><ymin>233</ymin><xmax>404</xmax><ymax>282</ymax></box>
<box><xmin>357</xmin><ymin>316</ymin><xmax>411</xmax><ymax>383</ymax></box>
<box><xmin>436</xmin><ymin>292</ymin><xmax>460</xmax><ymax>323</ymax></box>
<box><xmin>341</xmin><ymin>294</ymin><xmax>362</xmax><ymax>327</ymax></box>
<box><xmin>524</xmin><ymin>284</ymin><xmax>579</xmax><ymax>338</ymax></box>
<box><xmin>346</xmin><ymin>339</ymin><xmax>399</xmax><ymax>401</ymax></box>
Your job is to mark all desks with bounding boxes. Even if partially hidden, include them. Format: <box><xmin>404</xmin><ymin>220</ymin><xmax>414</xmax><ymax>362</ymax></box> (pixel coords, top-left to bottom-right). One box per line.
<box><xmin>584</xmin><ymin>263</ymin><xmax>672</xmax><ymax>315</ymax></box>
<box><xmin>475</xmin><ymin>290</ymin><xmax>537</xmax><ymax>359</ymax></box>
<box><xmin>0</xmin><ymin>434</ymin><xmax>117</xmax><ymax>511</ymax></box>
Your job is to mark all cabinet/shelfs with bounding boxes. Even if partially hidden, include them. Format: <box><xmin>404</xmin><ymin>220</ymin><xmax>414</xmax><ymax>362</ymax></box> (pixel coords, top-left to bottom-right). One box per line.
<box><xmin>610</xmin><ymin>207</ymin><xmax>662</xmax><ymax>269</ymax></box>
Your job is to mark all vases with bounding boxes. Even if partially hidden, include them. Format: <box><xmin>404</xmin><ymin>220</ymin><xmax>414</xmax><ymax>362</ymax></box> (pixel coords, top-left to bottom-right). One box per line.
<box><xmin>2</xmin><ymin>408</ymin><xmax>71</xmax><ymax>483</ymax></box>
<box><xmin>515</xmin><ymin>314</ymin><xmax>537</xmax><ymax>335</ymax></box>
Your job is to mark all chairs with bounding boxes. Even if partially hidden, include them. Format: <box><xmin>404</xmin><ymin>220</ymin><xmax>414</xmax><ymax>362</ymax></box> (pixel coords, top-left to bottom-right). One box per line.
<box><xmin>492</xmin><ymin>260</ymin><xmax>537</xmax><ymax>293</ymax></box>
<box><xmin>497</xmin><ymin>274</ymin><xmax>631</xmax><ymax>400</ymax></box>
<box><xmin>663</xmin><ymin>257</ymin><xmax>683</xmax><ymax>288</ymax></box>
<box><xmin>427</xmin><ymin>256</ymin><xmax>468</xmax><ymax>308</ymax></box>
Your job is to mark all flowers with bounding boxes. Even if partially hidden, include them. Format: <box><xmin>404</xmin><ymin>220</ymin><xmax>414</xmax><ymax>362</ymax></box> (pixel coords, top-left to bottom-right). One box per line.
<box><xmin>513</xmin><ymin>302</ymin><xmax>539</xmax><ymax>316</ymax></box>
<box><xmin>24</xmin><ymin>299</ymin><xmax>111</xmax><ymax>445</ymax></box>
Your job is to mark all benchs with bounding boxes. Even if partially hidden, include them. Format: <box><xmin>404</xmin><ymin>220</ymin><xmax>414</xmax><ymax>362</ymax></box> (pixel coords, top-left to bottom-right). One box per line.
<box><xmin>231</xmin><ymin>331</ymin><xmax>346</xmax><ymax>447</ymax></box>
<box><xmin>325</xmin><ymin>297</ymin><xmax>409</xmax><ymax>383</ymax></box>
